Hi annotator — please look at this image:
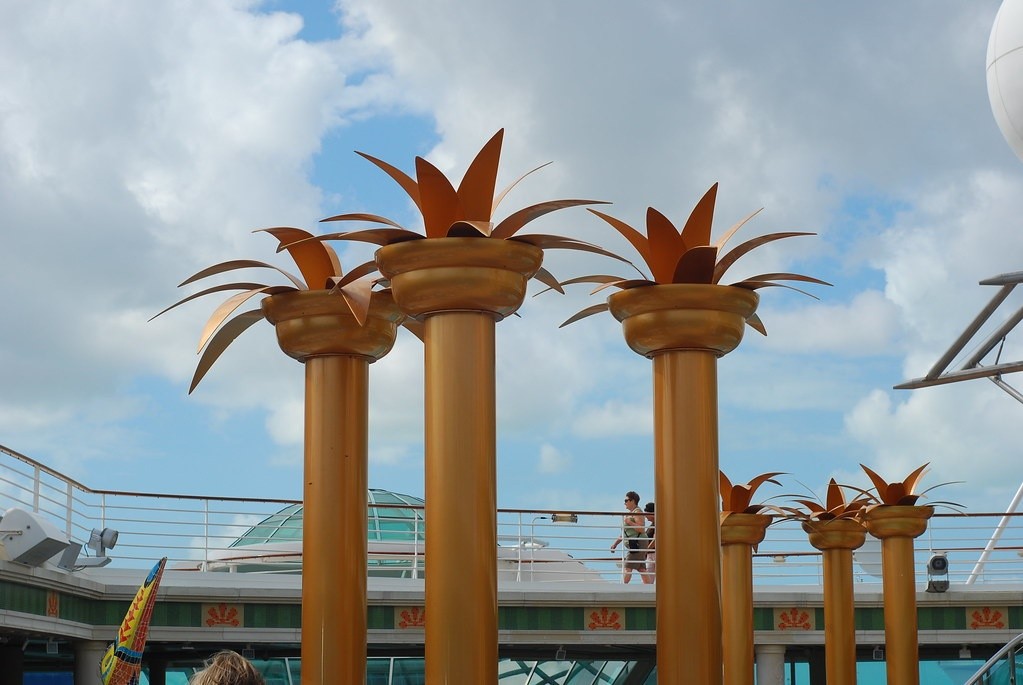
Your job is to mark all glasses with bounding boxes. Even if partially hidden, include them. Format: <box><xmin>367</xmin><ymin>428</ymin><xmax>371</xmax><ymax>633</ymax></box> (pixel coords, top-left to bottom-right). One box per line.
<box><xmin>625</xmin><ymin>500</ymin><xmax>630</xmax><ymax>503</ymax></box>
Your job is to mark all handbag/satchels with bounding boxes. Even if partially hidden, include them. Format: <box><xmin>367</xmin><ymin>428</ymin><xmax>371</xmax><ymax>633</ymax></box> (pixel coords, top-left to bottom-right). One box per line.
<box><xmin>629</xmin><ymin>540</ymin><xmax>639</xmax><ymax>553</ymax></box>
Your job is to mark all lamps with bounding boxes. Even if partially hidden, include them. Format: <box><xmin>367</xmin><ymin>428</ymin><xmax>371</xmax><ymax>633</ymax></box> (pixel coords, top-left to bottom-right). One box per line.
<box><xmin>873</xmin><ymin>645</ymin><xmax>884</xmax><ymax>660</ymax></box>
<box><xmin>925</xmin><ymin>555</ymin><xmax>950</xmax><ymax>593</ymax></box>
<box><xmin>46</xmin><ymin>636</ymin><xmax>58</xmax><ymax>654</ymax></box>
<box><xmin>555</xmin><ymin>644</ymin><xmax>566</xmax><ymax>660</ymax></box>
<box><xmin>243</xmin><ymin>644</ymin><xmax>255</xmax><ymax>659</ymax></box>
<box><xmin>75</xmin><ymin>526</ymin><xmax>119</xmax><ymax>568</ymax></box>
<box><xmin>959</xmin><ymin>645</ymin><xmax>971</xmax><ymax>659</ymax></box>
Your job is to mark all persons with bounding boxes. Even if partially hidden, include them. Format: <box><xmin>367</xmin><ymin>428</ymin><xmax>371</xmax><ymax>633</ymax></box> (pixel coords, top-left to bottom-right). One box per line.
<box><xmin>644</xmin><ymin>503</ymin><xmax>655</xmax><ymax>585</ymax></box>
<box><xmin>189</xmin><ymin>650</ymin><xmax>267</xmax><ymax>685</ymax></box>
<box><xmin>611</xmin><ymin>491</ymin><xmax>647</xmax><ymax>584</ymax></box>
